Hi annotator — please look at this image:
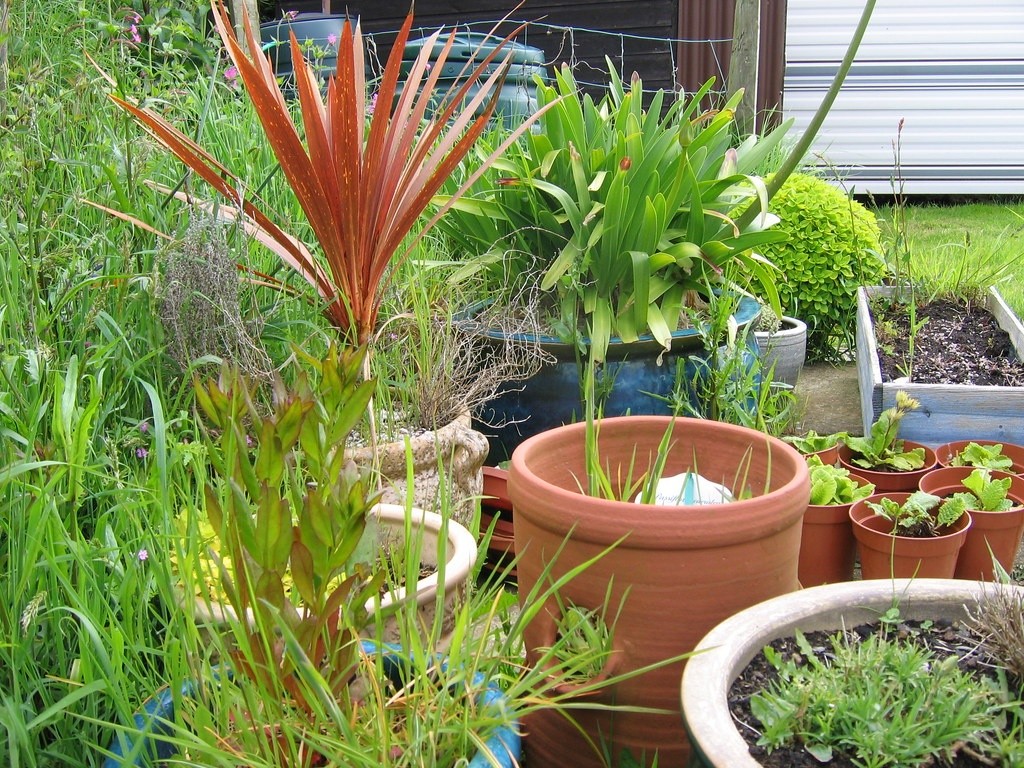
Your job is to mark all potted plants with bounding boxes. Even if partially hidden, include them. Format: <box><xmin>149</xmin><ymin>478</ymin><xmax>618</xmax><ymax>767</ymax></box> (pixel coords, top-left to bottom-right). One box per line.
<box><xmin>40</xmin><ymin>1</ymin><xmax>723</xmax><ymax>768</ymax></box>
<box><xmin>934</xmin><ymin>441</ymin><xmax>1024</xmax><ymax>479</ymax></box>
<box><xmin>838</xmin><ymin>389</ymin><xmax>938</xmax><ymax>494</ymax></box>
<box><xmin>504</xmin><ymin>298</ymin><xmax>811</xmax><ymax>768</ymax></box>
<box><xmin>918</xmin><ymin>467</ymin><xmax>1024</xmax><ymax>583</ymax></box>
<box><xmin>678</xmin><ymin>511</ymin><xmax>1024</xmax><ymax>768</ymax></box>
<box><xmin>806</xmin><ymin>120</ymin><xmax>1024</xmax><ymax>452</ymax></box>
<box><xmin>780</xmin><ymin>429</ymin><xmax>848</xmax><ymax>465</ymax></box>
<box><xmin>799</xmin><ymin>452</ymin><xmax>876</xmax><ymax>588</ymax></box>
<box><xmin>418</xmin><ymin>0</ymin><xmax>877</xmax><ymax>467</ymax></box>
<box><xmin>716</xmin><ymin>301</ymin><xmax>808</xmax><ymax>406</ymax></box>
<box><xmin>849</xmin><ymin>490</ymin><xmax>972</xmax><ymax>580</ymax></box>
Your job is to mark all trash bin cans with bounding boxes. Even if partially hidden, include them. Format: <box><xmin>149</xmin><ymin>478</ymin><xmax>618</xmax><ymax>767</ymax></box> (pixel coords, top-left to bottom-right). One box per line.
<box><xmin>259</xmin><ymin>12</ymin><xmax>371</xmax><ymax>101</ymax></box>
<box><xmin>389</xmin><ymin>31</ymin><xmax>548</xmax><ymax>134</ymax></box>
